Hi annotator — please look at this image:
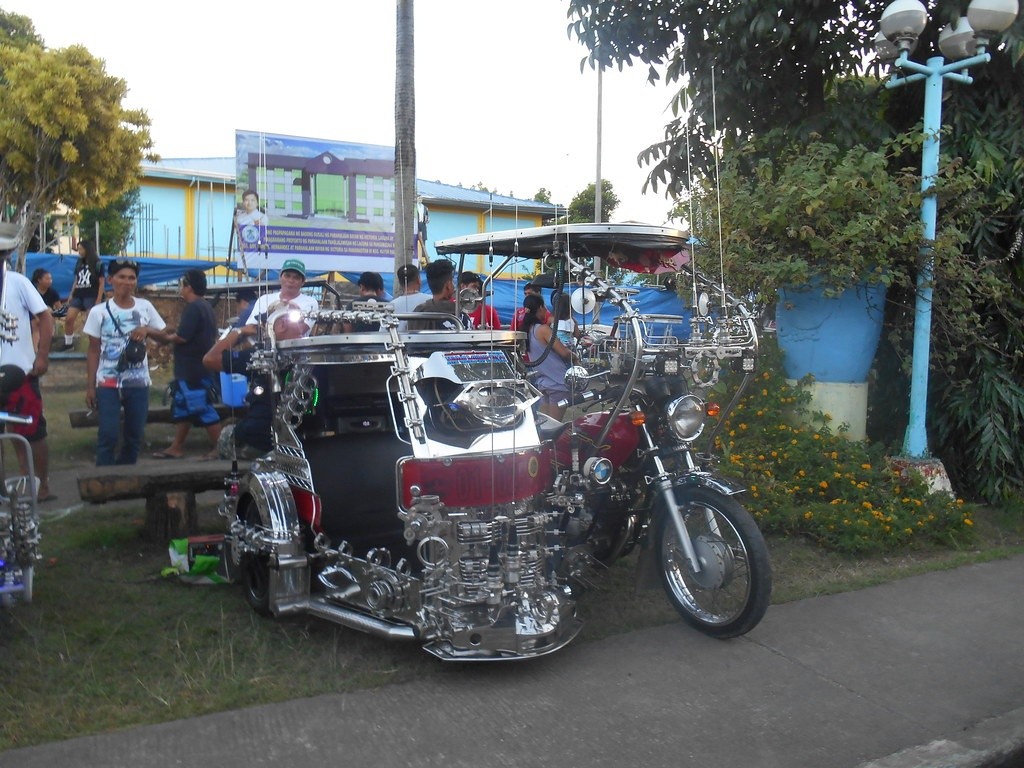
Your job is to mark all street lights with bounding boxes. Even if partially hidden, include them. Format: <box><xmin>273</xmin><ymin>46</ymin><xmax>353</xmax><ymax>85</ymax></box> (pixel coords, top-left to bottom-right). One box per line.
<box><xmin>875</xmin><ymin>0</ymin><xmax>1019</xmax><ymax>512</ymax></box>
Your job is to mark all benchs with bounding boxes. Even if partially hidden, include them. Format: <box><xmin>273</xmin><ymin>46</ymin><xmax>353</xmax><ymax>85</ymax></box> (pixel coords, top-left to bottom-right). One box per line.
<box><xmin>74</xmin><ymin>457</ymin><xmax>251</xmax><ymax>543</ymax></box>
<box><xmin>301</xmin><ymin>429</ymin><xmax>410</xmax><ymax>555</ymax></box>
<box><xmin>68</xmin><ymin>401</ymin><xmax>253</xmax><ymax>451</ymax></box>
<box><xmin>46</xmin><ymin>351</ymin><xmax>88</xmax><ymax>363</ymax></box>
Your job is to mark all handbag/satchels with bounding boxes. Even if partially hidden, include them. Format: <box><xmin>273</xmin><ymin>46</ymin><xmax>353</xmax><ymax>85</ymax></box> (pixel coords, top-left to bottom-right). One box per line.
<box><xmin>126</xmin><ymin>340</ymin><xmax>146</xmax><ymax>365</ymax></box>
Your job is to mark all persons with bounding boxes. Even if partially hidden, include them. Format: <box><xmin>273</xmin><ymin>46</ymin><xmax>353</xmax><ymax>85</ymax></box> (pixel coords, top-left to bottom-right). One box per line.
<box><xmin>85</xmin><ymin>258</ymin><xmax>167</xmax><ymax>467</ymax></box>
<box><xmin>450</xmin><ymin>271</ymin><xmax>502</xmax><ymax>329</ymax></box>
<box><xmin>341</xmin><ymin>272</ymin><xmax>391</xmax><ymax>333</ymax></box>
<box><xmin>0</xmin><ymin>236</ymin><xmax>58</xmax><ymax>502</ymax></box>
<box><xmin>227</xmin><ymin>288</ymin><xmax>258</xmax><ymax>349</ymax></box>
<box><xmin>237</xmin><ymin>190</ymin><xmax>269</xmax><ymax>268</ymax></box>
<box><xmin>57</xmin><ymin>240</ymin><xmax>106</xmax><ymax>352</ymax></box>
<box><xmin>389</xmin><ymin>263</ymin><xmax>434</xmax><ymax>332</ymax></box>
<box><xmin>245</xmin><ymin>258</ymin><xmax>319</xmax><ymax>347</ymax></box>
<box><xmin>516</xmin><ymin>294</ymin><xmax>581</xmax><ymax>422</ymax></box>
<box><xmin>28</xmin><ymin>267</ymin><xmax>62</xmax><ymax>355</ymax></box>
<box><xmin>509</xmin><ymin>283</ymin><xmax>551</xmax><ymax>408</ymax></box>
<box><xmin>202</xmin><ymin>300</ymin><xmax>300</xmax><ymax>518</ymax></box>
<box><xmin>546</xmin><ymin>290</ymin><xmax>581</xmax><ymax>369</ymax></box>
<box><xmin>151</xmin><ymin>270</ymin><xmax>223</xmax><ymax>460</ymax></box>
<box><xmin>402</xmin><ymin>259</ymin><xmax>472</xmax><ymax>329</ymax></box>
<box><xmin>417</xmin><ymin>195</ymin><xmax>430</xmax><ymax>258</ymax></box>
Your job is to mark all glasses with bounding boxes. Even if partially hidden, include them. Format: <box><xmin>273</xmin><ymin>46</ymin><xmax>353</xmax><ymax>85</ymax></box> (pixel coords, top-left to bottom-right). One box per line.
<box><xmin>115</xmin><ymin>258</ymin><xmax>137</xmax><ymax>267</ymax></box>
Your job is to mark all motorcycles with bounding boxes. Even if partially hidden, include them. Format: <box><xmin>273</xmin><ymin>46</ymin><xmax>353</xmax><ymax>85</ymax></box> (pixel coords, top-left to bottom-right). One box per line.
<box><xmin>217</xmin><ymin>222</ymin><xmax>772</xmax><ymax>661</ymax></box>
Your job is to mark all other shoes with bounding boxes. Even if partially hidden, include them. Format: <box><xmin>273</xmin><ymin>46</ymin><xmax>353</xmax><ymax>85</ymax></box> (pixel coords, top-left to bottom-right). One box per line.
<box><xmin>39</xmin><ymin>494</ymin><xmax>58</xmax><ymax>502</ymax></box>
<box><xmin>56</xmin><ymin>343</ymin><xmax>74</xmax><ymax>352</ymax></box>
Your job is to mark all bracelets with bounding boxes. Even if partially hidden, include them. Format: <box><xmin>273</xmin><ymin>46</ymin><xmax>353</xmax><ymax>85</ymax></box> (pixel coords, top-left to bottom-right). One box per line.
<box><xmin>231</xmin><ymin>327</ymin><xmax>241</xmax><ymax>336</ymax></box>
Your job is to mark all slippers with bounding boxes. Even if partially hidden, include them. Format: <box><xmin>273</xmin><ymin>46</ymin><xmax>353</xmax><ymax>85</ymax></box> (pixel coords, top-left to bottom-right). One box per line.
<box><xmin>199</xmin><ymin>454</ymin><xmax>224</xmax><ymax>461</ymax></box>
<box><xmin>153</xmin><ymin>450</ymin><xmax>176</xmax><ymax>459</ymax></box>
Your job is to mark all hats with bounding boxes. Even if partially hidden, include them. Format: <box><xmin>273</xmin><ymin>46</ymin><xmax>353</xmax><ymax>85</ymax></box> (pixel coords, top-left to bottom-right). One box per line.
<box><xmin>280</xmin><ymin>259</ymin><xmax>305</xmax><ymax>279</ymax></box>
